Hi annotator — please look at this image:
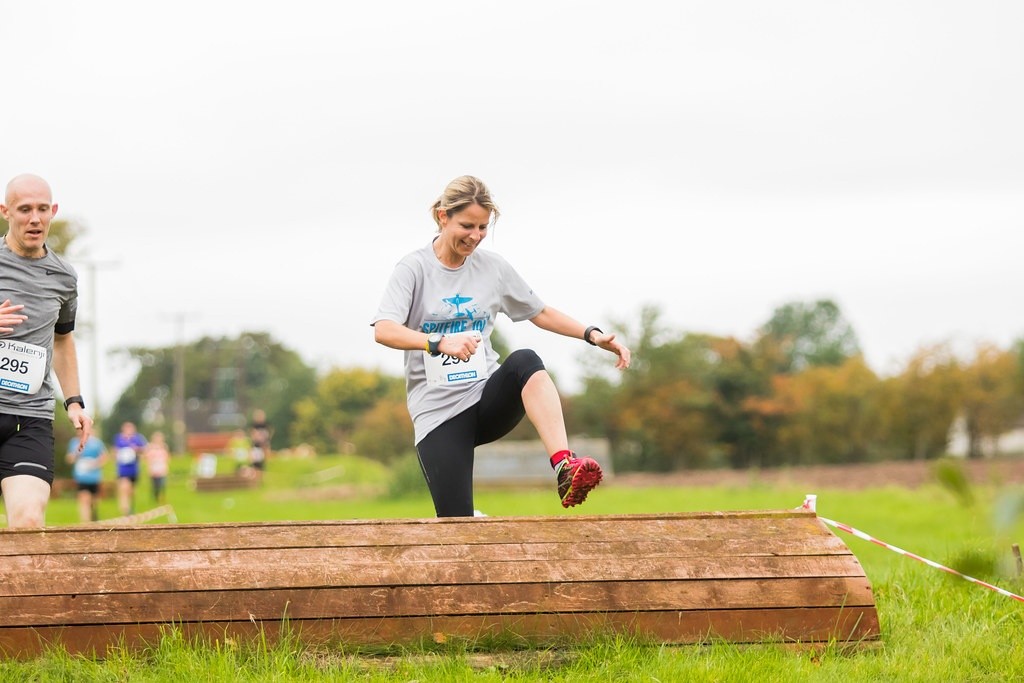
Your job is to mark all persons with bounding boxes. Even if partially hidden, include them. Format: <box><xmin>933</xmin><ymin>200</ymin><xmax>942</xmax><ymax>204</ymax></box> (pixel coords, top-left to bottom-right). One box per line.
<box><xmin>145</xmin><ymin>431</ymin><xmax>170</xmax><ymax>498</ymax></box>
<box><xmin>65</xmin><ymin>420</ymin><xmax>107</xmax><ymax>522</ymax></box>
<box><xmin>111</xmin><ymin>421</ymin><xmax>148</xmax><ymax>515</ymax></box>
<box><xmin>0</xmin><ymin>173</ymin><xmax>94</xmax><ymax>529</ymax></box>
<box><xmin>370</xmin><ymin>175</ymin><xmax>631</xmax><ymax>517</ymax></box>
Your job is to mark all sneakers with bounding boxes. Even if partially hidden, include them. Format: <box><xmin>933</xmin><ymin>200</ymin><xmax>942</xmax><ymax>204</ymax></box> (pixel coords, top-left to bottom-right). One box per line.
<box><xmin>556</xmin><ymin>456</ymin><xmax>603</xmax><ymax>507</ymax></box>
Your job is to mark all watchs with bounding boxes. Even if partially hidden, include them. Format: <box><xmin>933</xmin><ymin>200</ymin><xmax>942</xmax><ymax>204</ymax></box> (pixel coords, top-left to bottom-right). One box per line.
<box><xmin>64</xmin><ymin>395</ymin><xmax>85</xmax><ymax>410</ymax></box>
<box><xmin>428</xmin><ymin>333</ymin><xmax>444</xmax><ymax>356</ymax></box>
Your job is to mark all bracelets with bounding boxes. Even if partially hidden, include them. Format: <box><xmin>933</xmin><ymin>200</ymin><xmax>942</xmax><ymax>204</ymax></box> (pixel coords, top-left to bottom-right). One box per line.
<box><xmin>584</xmin><ymin>326</ymin><xmax>603</xmax><ymax>346</ymax></box>
<box><xmin>425</xmin><ymin>340</ymin><xmax>430</xmax><ymax>354</ymax></box>
